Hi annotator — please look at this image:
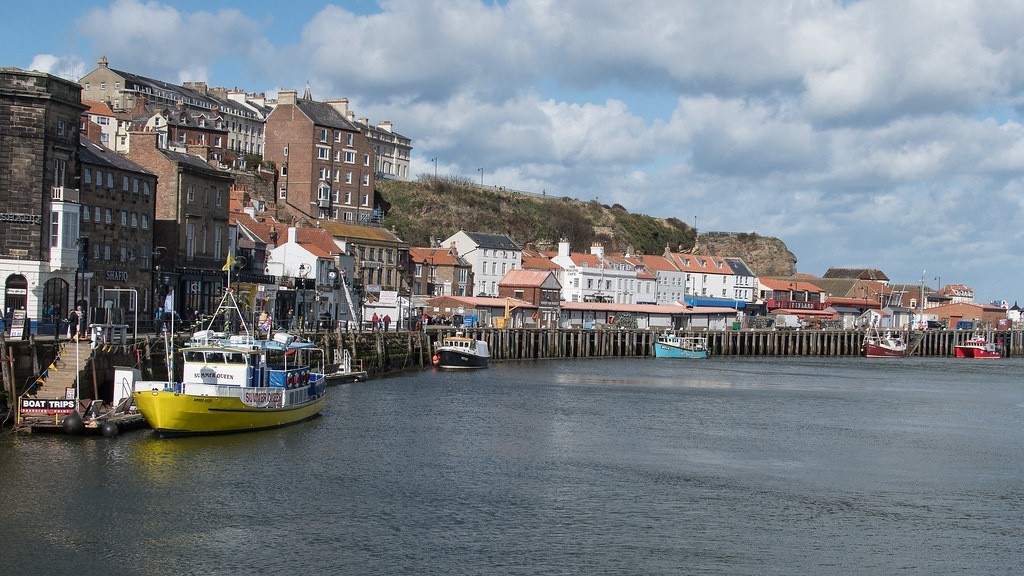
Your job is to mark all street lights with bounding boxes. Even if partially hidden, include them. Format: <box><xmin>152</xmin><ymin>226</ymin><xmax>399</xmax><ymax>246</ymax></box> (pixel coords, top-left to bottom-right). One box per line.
<box><xmin>232</xmin><ymin>256</ymin><xmax>248</xmax><ymax>335</ymax></box>
<box><xmin>861</xmin><ymin>286</ymin><xmax>868</xmax><ymax>311</ymax></box>
<box><xmin>151</xmin><ymin>246</ymin><xmax>168</xmax><ymax>335</ymax></box>
<box><xmin>423</xmin><ymin>256</ymin><xmax>438</xmax><ymax>298</ymax></box>
<box><xmin>934</xmin><ymin>277</ymin><xmax>940</xmax><ymax>290</ymax></box>
<box><xmin>74</xmin><ymin>237</ymin><xmax>87</xmax><ymax>338</ymax></box>
<box><xmin>790</xmin><ymin>282</ymin><xmax>797</xmax><ymax>300</ymax></box>
<box><xmin>406</xmin><ymin>276</ymin><xmax>413</xmax><ymax>331</ymax></box>
<box><xmin>299</xmin><ymin>262</ymin><xmax>312</xmax><ymax>329</ymax></box>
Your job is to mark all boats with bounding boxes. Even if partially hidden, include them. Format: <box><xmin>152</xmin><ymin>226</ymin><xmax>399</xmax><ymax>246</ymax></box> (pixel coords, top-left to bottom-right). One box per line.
<box><xmin>954</xmin><ymin>331</ymin><xmax>1002</xmax><ymax>358</ymax></box>
<box><xmin>862</xmin><ymin>321</ymin><xmax>907</xmax><ymax>357</ymax></box>
<box><xmin>319</xmin><ymin>348</ymin><xmax>369</xmax><ymax>384</ymax></box>
<box><xmin>654</xmin><ymin>334</ymin><xmax>711</xmax><ymax>359</ymax></box>
<box><xmin>433</xmin><ymin>337</ymin><xmax>491</xmax><ymax>369</ymax></box>
<box><xmin>132</xmin><ymin>246</ymin><xmax>326</xmax><ymax>438</ymax></box>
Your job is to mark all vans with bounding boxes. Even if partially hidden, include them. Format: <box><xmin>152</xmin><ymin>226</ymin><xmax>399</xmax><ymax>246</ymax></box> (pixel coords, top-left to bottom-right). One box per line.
<box><xmin>776</xmin><ymin>314</ymin><xmax>803</xmax><ymax>330</ymax></box>
<box><xmin>928</xmin><ymin>321</ymin><xmax>945</xmax><ymax>331</ymax></box>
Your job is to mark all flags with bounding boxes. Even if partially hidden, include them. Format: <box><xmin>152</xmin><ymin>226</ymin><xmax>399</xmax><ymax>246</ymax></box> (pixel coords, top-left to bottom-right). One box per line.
<box><xmin>222</xmin><ymin>253</ymin><xmax>237</xmax><ymax>272</ymax></box>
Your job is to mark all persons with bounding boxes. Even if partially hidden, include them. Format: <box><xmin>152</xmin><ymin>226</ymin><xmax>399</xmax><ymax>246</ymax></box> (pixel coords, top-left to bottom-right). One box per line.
<box><xmin>422</xmin><ymin>312</ymin><xmax>453</xmax><ymax>327</ymax></box>
<box><xmin>371</xmin><ymin>313</ymin><xmax>391</xmax><ymax>331</ymax></box>
<box><xmin>63</xmin><ymin>309</ymin><xmax>79</xmax><ymax>340</ymax></box>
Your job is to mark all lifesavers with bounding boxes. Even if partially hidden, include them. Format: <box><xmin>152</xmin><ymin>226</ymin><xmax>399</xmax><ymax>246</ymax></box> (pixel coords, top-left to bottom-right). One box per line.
<box><xmin>307</xmin><ymin>370</ymin><xmax>310</xmax><ymax>385</ymax></box>
<box><xmin>294</xmin><ymin>373</ymin><xmax>300</xmax><ymax>387</ymax></box>
<box><xmin>531</xmin><ymin>312</ymin><xmax>537</xmax><ymax>319</ymax></box>
<box><xmin>918</xmin><ymin>324</ymin><xmax>923</xmax><ymax>330</ymax></box>
<box><xmin>301</xmin><ymin>371</ymin><xmax>306</xmax><ymax>386</ymax></box>
<box><xmin>287</xmin><ymin>372</ymin><xmax>293</xmax><ymax>387</ymax></box>
